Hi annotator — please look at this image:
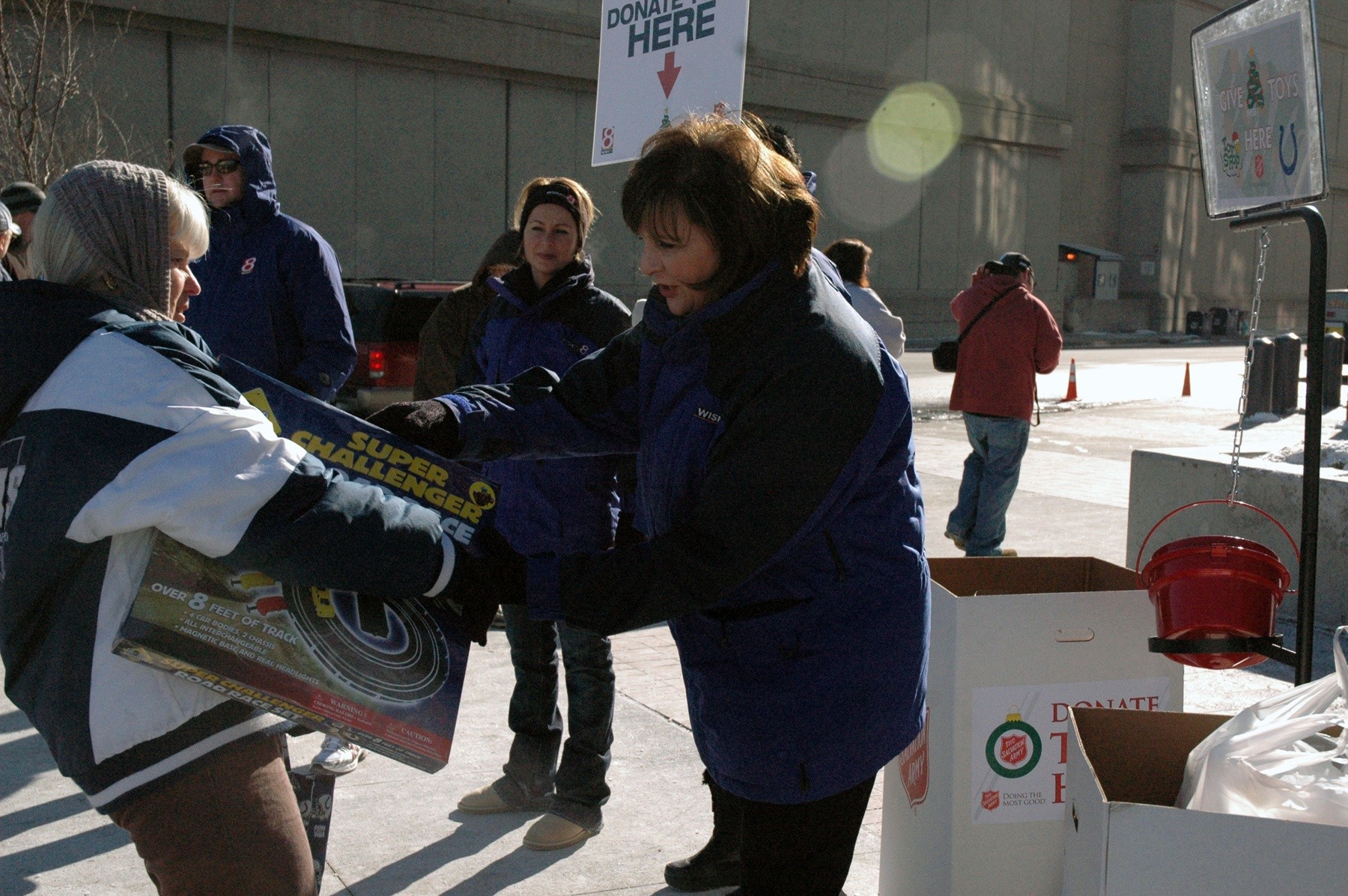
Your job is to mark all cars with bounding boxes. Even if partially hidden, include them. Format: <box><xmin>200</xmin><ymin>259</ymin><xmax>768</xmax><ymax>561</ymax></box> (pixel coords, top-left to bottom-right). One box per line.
<box><xmin>336</xmin><ymin>275</ymin><xmax>475</xmax><ymax>407</ymax></box>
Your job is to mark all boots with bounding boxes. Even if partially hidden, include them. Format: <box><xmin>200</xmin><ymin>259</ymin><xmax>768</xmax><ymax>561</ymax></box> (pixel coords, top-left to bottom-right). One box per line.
<box><xmin>663</xmin><ymin>769</ymin><xmax>750</xmax><ymax>892</ymax></box>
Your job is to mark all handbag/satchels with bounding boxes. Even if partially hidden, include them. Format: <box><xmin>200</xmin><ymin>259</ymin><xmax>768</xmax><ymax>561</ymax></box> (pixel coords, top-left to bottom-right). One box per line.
<box><xmin>932</xmin><ymin>341</ymin><xmax>960</xmax><ymax>373</ymax></box>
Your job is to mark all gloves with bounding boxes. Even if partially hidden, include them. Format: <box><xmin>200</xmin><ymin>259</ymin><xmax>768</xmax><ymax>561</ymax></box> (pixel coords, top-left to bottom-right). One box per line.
<box><xmin>560</xmin><ymin>551</ymin><xmax>606</xmax><ymax>638</ymax></box>
<box><xmin>366</xmin><ymin>401</ymin><xmax>465</xmax><ymax>457</ymax></box>
<box><xmin>450</xmin><ymin>535</ymin><xmax>527</xmax><ymax>604</ymax></box>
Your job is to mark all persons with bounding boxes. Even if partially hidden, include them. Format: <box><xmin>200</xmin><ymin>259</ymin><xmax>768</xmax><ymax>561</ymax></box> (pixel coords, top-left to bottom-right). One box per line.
<box><xmin>412</xmin><ymin>176</ymin><xmax>632</xmax><ymax>850</ymax></box>
<box><xmin>823</xmin><ymin>238</ymin><xmax>907</xmax><ymax>360</ymax></box>
<box><xmin>0</xmin><ymin>182</ymin><xmax>47</xmax><ymax>282</ymax></box>
<box><xmin>944</xmin><ymin>252</ymin><xmax>1062</xmax><ymax>557</ymax></box>
<box><xmin>365</xmin><ymin>109</ymin><xmax>928</xmax><ymax>895</ymax></box>
<box><xmin>0</xmin><ymin>158</ymin><xmax>501</xmax><ymax>896</ymax></box>
<box><xmin>182</xmin><ymin>125</ymin><xmax>358</xmax><ymax>404</ymax></box>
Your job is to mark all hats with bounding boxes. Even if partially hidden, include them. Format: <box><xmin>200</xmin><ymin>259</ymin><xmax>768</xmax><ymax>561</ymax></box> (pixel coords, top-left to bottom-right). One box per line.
<box><xmin>0</xmin><ymin>180</ymin><xmax>46</xmax><ymax>215</ymax></box>
<box><xmin>0</xmin><ymin>202</ymin><xmax>23</xmax><ymax>236</ymax></box>
<box><xmin>988</xmin><ymin>251</ymin><xmax>1034</xmax><ymax>278</ymax></box>
<box><xmin>181</xmin><ymin>143</ymin><xmax>233</xmax><ymax>164</ymax></box>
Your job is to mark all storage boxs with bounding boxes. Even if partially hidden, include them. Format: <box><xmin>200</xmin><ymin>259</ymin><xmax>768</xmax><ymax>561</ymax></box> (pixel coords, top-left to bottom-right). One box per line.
<box><xmin>880</xmin><ymin>556</ymin><xmax>1184</xmax><ymax>896</ymax></box>
<box><xmin>1062</xmin><ymin>706</ymin><xmax>1348</xmax><ymax>896</ymax></box>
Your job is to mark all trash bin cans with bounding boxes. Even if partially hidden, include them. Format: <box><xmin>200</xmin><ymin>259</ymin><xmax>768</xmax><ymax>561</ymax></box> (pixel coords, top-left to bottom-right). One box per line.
<box><xmin>1210</xmin><ymin>307</ymin><xmax>1228</xmax><ymax>336</ymax></box>
<box><xmin>1186</xmin><ymin>311</ymin><xmax>1203</xmax><ymax>335</ymax></box>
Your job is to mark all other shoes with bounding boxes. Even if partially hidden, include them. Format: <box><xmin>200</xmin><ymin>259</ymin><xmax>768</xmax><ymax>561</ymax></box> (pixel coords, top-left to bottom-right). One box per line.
<box><xmin>1003</xmin><ymin>548</ymin><xmax>1019</xmax><ymax>557</ymax></box>
<box><xmin>948</xmin><ymin>533</ymin><xmax>967</xmax><ymax>551</ymax></box>
<box><xmin>457</xmin><ymin>786</ymin><xmax>519</xmax><ymax>813</ymax></box>
<box><xmin>523</xmin><ymin>813</ymin><xmax>592</xmax><ymax>849</ymax></box>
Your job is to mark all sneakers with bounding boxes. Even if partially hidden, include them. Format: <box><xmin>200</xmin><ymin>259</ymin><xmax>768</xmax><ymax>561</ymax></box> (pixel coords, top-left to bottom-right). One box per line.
<box><xmin>309</xmin><ymin>729</ymin><xmax>368</xmax><ymax>775</ymax></box>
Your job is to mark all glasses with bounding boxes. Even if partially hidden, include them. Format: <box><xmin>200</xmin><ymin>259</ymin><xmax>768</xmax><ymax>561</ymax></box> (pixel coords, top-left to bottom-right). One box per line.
<box><xmin>195</xmin><ymin>158</ymin><xmax>241</xmax><ymax>176</ymax></box>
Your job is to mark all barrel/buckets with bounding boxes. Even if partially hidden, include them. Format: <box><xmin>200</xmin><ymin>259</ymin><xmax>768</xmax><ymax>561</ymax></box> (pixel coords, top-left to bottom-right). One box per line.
<box><xmin>1135</xmin><ymin>497</ymin><xmax>1303</xmax><ymax>670</ymax></box>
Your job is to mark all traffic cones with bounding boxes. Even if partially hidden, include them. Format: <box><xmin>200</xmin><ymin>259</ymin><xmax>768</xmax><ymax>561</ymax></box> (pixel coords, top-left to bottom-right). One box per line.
<box><xmin>1179</xmin><ymin>362</ymin><xmax>1192</xmax><ymax>396</ymax></box>
<box><xmin>1060</xmin><ymin>359</ymin><xmax>1082</xmax><ymax>403</ymax></box>
